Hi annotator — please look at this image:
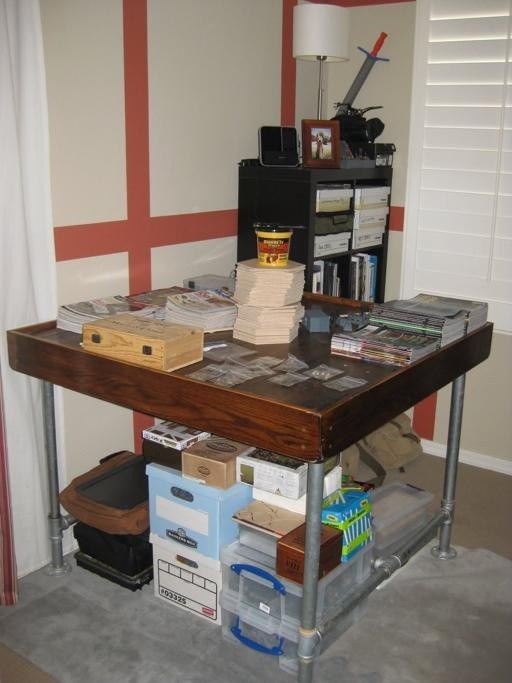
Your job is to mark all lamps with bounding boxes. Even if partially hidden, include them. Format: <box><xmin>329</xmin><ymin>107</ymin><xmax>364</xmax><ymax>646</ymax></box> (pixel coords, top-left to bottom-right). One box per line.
<box><xmin>292</xmin><ymin>2</ymin><xmax>350</xmax><ymax>119</ymax></box>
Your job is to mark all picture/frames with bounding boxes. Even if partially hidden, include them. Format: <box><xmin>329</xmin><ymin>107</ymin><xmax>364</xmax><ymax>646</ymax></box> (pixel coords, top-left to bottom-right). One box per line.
<box><xmin>301</xmin><ymin>118</ymin><xmax>341</xmax><ymax>168</ymax></box>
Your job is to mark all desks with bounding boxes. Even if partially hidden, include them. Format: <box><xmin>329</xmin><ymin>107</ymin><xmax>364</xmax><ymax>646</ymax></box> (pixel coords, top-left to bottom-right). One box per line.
<box><xmin>6</xmin><ymin>285</ymin><xmax>494</xmax><ymax>682</ymax></box>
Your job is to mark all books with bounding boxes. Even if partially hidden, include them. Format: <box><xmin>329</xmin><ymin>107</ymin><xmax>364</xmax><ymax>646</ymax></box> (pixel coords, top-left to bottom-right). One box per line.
<box><xmin>312</xmin><ymin>252</ymin><xmax>379</xmax><ymax>304</ymax></box>
<box><xmin>330</xmin><ymin>291</ymin><xmax>489</xmax><ymax>369</ymax></box>
<box><xmin>53</xmin><ymin>283</ymin><xmax>238</xmax><ymax>338</ymax></box>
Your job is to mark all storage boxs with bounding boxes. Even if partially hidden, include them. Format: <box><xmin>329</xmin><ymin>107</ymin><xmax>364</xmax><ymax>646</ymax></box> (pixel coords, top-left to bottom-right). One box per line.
<box><xmin>141</xmin><ymin>417</ymin><xmax>437</xmax><ymax>675</ymax></box>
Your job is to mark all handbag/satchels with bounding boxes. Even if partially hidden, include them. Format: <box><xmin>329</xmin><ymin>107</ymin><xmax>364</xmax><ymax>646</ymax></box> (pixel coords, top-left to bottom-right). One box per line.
<box><xmin>340</xmin><ymin>411</ymin><xmax>423</xmax><ymax>489</ymax></box>
<box><xmin>58</xmin><ymin>450</ymin><xmax>150</xmax><ymax>536</ymax></box>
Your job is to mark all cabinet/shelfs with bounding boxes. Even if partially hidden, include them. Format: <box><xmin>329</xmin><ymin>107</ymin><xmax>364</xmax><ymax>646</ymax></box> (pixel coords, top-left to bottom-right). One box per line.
<box><xmin>235</xmin><ymin>164</ymin><xmax>393</xmax><ymax>304</ymax></box>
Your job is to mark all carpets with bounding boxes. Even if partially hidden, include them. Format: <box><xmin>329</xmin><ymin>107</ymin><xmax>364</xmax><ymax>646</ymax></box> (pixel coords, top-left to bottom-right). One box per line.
<box><xmin>1</xmin><ymin>535</ymin><xmax>511</xmax><ymax>682</ymax></box>
